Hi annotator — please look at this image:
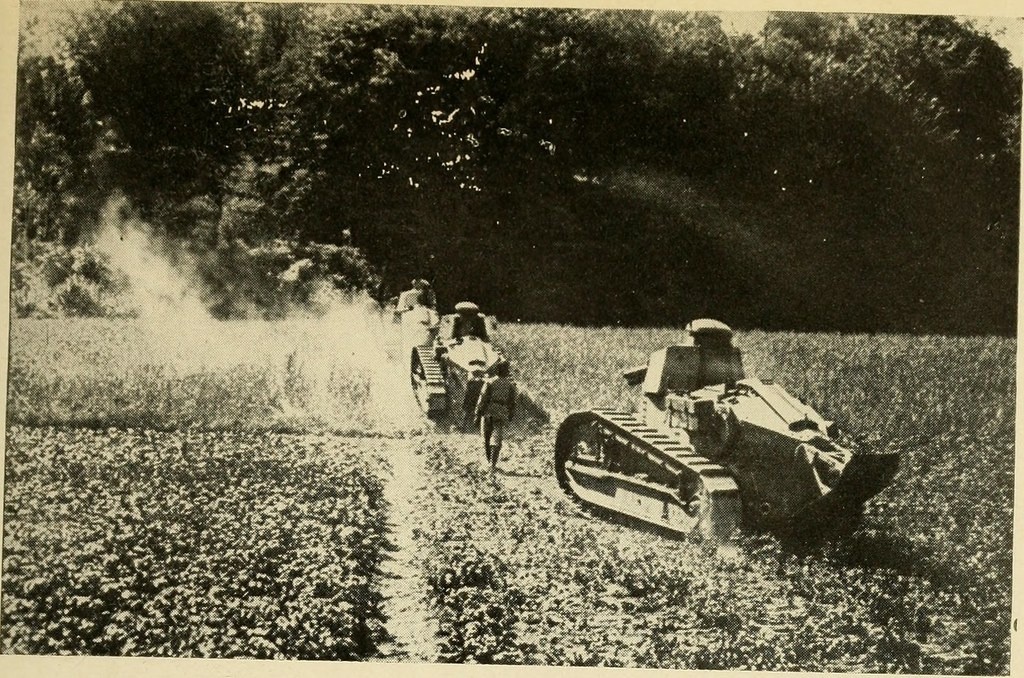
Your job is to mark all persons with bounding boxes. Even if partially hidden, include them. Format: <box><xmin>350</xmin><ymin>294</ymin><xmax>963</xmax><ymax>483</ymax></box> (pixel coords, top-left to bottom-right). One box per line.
<box><xmin>473</xmin><ymin>361</ymin><xmax>516</xmax><ymax>467</ymax></box>
<box><xmin>413</xmin><ymin>279</ymin><xmax>432</xmax><ymax>305</ymax></box>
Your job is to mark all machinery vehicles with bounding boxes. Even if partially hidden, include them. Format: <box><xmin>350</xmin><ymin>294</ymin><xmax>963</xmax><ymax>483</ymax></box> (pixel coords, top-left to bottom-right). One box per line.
<box><xmin>408</xmin><ymin>299</ymin><xmax>518</xmax><ymax>420</ymax></box>
<box><xmin>551</xmin><ymin>316</ymin><xmax>902</xmax><ymax>547</ymax></box>
